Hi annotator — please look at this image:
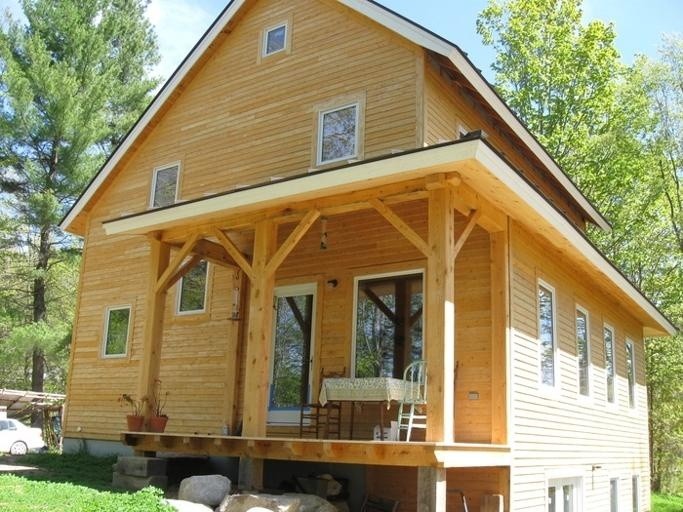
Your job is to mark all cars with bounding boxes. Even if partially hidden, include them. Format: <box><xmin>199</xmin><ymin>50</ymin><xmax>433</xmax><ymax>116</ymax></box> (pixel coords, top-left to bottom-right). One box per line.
<box><xmin>0</xmin><ymin>418</ymin><xmax>49</xmax><ymax>455</ymax></box>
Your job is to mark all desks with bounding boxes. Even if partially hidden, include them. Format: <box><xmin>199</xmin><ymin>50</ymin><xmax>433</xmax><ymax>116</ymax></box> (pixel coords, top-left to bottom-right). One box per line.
<box><xmin>321</xmin><ymin>376</ymin><xmax>419</xmax><ymax>441</ymax></box>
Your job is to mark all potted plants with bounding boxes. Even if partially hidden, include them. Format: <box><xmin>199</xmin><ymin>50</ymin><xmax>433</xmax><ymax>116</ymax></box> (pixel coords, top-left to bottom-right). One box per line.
<box><xmin>121</xmin><ymin>379</ymin><xmax>168</xmax><ymax>433</ymax></box>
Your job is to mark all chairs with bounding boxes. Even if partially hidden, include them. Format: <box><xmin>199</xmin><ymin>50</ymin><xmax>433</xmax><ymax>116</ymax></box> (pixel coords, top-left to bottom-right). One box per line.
<box><xmin>397</xmin><ymin>361</ymin><xmax>427</xmax><ymax>443</ymax></box>
<box><xmin>299</xmin><ymin>368</ymin><xmax>346</xmax><ymax>439</ymax></box>
